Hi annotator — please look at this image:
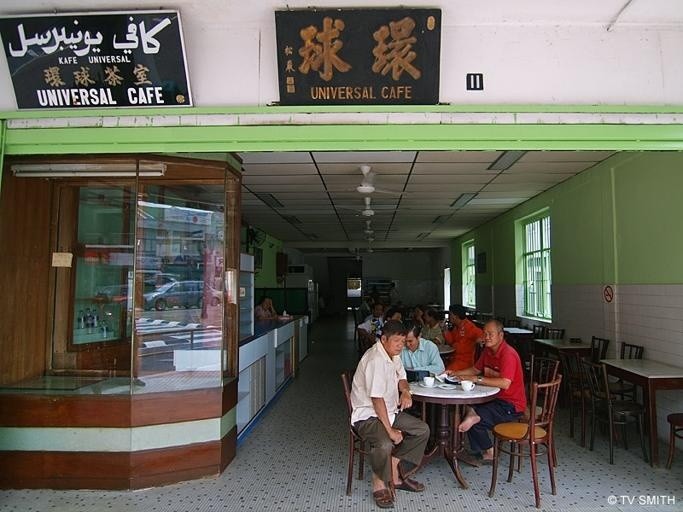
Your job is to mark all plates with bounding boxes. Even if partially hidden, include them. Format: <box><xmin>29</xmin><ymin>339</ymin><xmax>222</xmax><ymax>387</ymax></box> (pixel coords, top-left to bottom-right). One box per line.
<box><xmin>418</xmin><ymin>383</ymin><xmax>457</xmax><ymax>390</ymax></box>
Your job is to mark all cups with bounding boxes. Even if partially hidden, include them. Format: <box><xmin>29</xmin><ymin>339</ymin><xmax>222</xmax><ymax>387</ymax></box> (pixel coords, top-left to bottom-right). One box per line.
<box><xmin>423</xmin><ymin>376</ymin><xmax>435</xmax><ymax>387</ymax></box>
<box><xmin>461</xmin><ymin>381</ymin><xmax>476</xmax><ymax>391</ymax></box>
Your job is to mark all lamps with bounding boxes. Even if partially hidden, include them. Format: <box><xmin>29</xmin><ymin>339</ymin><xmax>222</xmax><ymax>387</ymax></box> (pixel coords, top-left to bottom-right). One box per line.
<box><xmin>10</xmin><ymin>162</ymin><xmax>168</xmax><ymax>180</ymax></box>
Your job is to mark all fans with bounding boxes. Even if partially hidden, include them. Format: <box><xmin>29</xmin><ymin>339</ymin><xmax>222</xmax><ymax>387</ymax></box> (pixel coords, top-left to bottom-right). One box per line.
<box><xmin>323</xmin><ymin>164</ymin><xmax>406</xmax><ymax>262</ymax></box>
<box><xmin>246</xmin><ymin>226</ymin><xmax>266</xmax><ymax>248</ymax></box>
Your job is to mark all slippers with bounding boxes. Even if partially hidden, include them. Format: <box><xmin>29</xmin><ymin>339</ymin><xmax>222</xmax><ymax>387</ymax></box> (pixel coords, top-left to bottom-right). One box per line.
<box><xmin>393</xmin><ymin>479</ymin><xmax>424</xmax><ymax>490</ymax></box>
<box><xmin>472</xmin><ymin>454</ymin><xmax>494</xmax><ymax>466</ymax></box>
<box><xmin>374</xmin><ymin>488</ymin><xmax>394</xmax><ymax>507</ymax></box>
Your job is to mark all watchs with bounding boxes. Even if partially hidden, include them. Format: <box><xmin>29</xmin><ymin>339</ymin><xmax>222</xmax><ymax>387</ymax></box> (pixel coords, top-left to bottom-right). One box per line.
<box><xmin>476</xmin><ymin>375</ymin><xmax>484</xmax><ymax>384</ymax></box>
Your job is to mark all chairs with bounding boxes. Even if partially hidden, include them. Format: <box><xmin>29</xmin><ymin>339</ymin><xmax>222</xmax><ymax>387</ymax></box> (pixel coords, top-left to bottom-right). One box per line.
<box><xmin>341</xmin><ymin>302</ymin><xmax>683</xmax><ymax>508</ymax></box>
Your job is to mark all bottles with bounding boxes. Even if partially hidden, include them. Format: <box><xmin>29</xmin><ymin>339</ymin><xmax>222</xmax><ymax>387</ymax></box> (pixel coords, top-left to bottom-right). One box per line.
<box><xmin>77</xmin><ymin>307</ymin><xmax>97</xmax><ymax>329</ymax></box>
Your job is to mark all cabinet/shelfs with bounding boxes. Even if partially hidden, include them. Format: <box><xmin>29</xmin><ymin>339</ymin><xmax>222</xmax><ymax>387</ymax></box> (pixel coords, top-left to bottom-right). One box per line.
<box><xmin>0</xmin><ymin>152</ymin><xmax>242</xmax><ymax>493</ymax></box>
<box><xmin>240</xmin><ymin>253</ymin><xmax>255</xmax><ymax>343</ymax></box>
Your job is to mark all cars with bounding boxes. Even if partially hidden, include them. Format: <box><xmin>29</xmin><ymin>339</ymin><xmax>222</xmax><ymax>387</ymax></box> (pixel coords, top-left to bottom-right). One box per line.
<box><xmin>95</xmin><ymin>269</ymin><xmax>224</xmax><ymax>312</ymax></box>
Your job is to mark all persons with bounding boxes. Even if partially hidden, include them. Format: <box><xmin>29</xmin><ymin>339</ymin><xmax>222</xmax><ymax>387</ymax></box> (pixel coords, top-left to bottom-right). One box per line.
<box><xmin>255</xmin><ymin>295</ymin><xmax>277</xmax><ymax>321</ymax></box>
<box><xmin>359</xmin><ymin>283</ymin><xmax>445</xmax><ymax>346</ymax></box>
<box><xmin>350</xmin><ymin>319</ymin><xmax>430</xmax><ymax>509</ymax></box>
<box><xmin>440</xmin><ymin>305</ymin><xmax>486</xmax><ymax>375</ymax></box>
<box><xmin>444</xmin><ymin>321</ymin><xmax>527</xmax><ymax>466</ymax></box>
<box><xmin>399</xmin><ymin>320</ymin><xmax>445</xmax><ymax>457</ymax></box>
